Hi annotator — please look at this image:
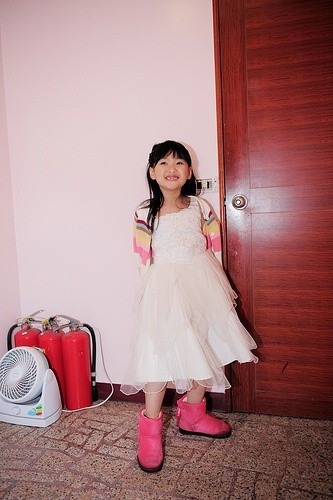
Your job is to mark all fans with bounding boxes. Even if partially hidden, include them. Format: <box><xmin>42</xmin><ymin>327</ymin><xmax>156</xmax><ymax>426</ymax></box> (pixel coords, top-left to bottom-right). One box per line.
<box><xmin>0</xmin><ymin>346</ymin><xmax>62</xmax><ymax>429</ymax></box>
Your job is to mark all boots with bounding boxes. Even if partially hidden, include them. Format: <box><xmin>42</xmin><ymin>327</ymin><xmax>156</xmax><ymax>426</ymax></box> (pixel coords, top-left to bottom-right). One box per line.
<box><xmin>177</xmin><ymin>393</ymin><xmax>232</xmax><ymax>439</ymax></box>
<box><xmin>137</xmin><ymin>408</ymin><xmax>163</xmax><ymax>473</ymax></box>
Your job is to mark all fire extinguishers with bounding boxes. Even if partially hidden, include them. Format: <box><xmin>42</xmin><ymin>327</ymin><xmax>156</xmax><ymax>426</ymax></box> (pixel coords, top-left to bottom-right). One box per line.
<box><xmin>56</xmin><ymin>316</ymin><xmax>97</xmax><ymax>411</ymax></box>
<box><xmin>7</xmin><ymin>309</ymin><xmax>45</xmax><ymax>351</ymax></box>
<box><xmin>37</xmin><ymin>315</ymin><xmax>66</xmax><ymax>408</ymax></box>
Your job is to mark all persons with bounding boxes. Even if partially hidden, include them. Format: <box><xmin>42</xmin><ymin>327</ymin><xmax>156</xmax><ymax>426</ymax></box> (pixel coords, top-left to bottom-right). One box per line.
<box><xmin>121</xmin><ymin>140</ymin><xmax>259</xmax><ymax>472</ymax></box>
